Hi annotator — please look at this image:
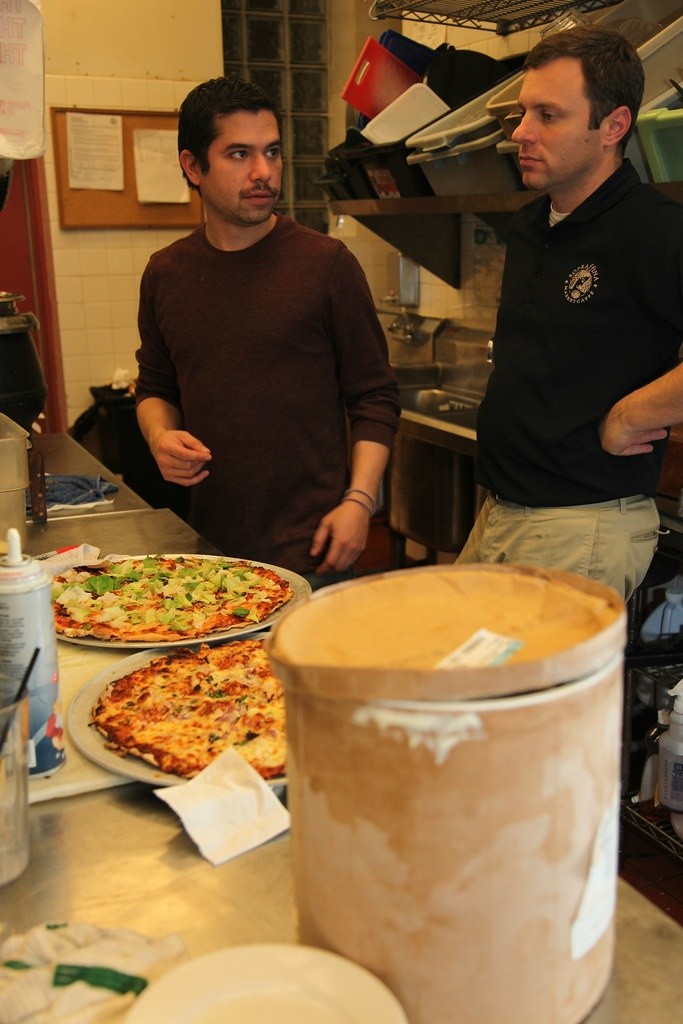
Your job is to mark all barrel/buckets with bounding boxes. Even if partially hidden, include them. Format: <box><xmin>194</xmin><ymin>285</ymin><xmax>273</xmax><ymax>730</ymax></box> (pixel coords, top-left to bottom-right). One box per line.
<box><xmin>264</xmin><ymin>560</ymin><xmax>629</xmax><ymax>1024</ymax></box>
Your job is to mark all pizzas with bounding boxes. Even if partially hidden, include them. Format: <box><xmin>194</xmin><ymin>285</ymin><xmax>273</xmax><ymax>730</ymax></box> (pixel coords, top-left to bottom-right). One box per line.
<box><xmin>52</xmin><ymin>553</ymin><xmax>289</xmax><ymax>642</ymax></box>
<box><xmin>93</xmin><ymin>641</ymin><xmax>285</xmax><ymax>778</ymax></box>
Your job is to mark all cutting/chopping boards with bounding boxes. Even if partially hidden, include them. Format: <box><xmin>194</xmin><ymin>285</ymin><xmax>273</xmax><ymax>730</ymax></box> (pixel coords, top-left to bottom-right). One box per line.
<box><xmin>378</xmin><ymin>28</ymin><xmax>433</xmax><ymax>77</ymax></box>
<box><xmin>341</xmin><ymin>35</ymin><xmax>422</xmax><ymax>120</ymax></box>
<box><xmin>359</xmin><ymin>82</ymin><xmax>451</xmax><ymax>145</ymax></box>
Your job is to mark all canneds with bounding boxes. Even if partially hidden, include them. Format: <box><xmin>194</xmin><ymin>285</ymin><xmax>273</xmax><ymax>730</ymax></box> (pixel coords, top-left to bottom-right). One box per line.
<box><xmin>0</xmin><ymin>529</ymin><xmax>71</xmax><ymax>779</ymax></box>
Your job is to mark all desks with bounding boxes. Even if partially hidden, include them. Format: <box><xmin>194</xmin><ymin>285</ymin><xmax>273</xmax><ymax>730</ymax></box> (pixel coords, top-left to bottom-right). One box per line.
<box><xmin>22</xmin><ymin>431</ymin><xmax>154</xmax><ymax>522</ymax></box>
<box><xmin>1</xmin><ymin>505</ymin><xmax>683</xmax><ymax>1024</ymax></box>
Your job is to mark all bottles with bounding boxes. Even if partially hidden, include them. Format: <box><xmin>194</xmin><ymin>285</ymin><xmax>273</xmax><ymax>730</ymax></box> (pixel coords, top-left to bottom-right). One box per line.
<box><xmin>1</xmin><ymin>527</ymin><xmax>65</xmax><ymax>780</ymax></box>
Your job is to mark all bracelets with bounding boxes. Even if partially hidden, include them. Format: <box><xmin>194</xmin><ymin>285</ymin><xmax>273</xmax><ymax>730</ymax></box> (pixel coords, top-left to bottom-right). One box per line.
<box><xmin>341</xmin><ymin>489</ymin><xmax>377</xmax><ymax>518</ymax></box>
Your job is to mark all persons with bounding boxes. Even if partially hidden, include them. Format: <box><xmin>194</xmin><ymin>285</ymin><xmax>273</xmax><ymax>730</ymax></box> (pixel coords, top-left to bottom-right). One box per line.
<box><xmin>451</xmin><ymin>26</ymin><xmax>682</xmax><ymax>600</ymax></box>
<box><xmin>134</xmin><ymin>70</ymin><xmax>401</xmax><ymax>593</ymax></box>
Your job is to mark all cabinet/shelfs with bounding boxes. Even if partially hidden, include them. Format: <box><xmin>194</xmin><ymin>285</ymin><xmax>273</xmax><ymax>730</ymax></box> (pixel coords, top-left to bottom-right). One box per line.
<box><xmin>323</xmin><ymin>0</ymin><xmax>683</xmax><ymax>288</ymax></box>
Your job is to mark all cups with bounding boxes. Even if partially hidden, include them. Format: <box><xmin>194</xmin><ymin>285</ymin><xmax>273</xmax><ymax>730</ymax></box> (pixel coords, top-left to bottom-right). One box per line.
<box><xmin>0</xmin><ymin>674</ymin><xmax>32</xmax><ymax>888</ymax></box>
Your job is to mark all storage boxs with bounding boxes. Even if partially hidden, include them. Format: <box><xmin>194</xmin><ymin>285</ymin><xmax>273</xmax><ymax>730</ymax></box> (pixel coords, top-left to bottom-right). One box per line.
<box><xmin>315</xmin><ymin>29</ymin><xmax>683</xmax><ymax>198</ymax></box>
<box><xmin>259</xmin><ymin>563</ymin><xmax>627</xmax><ymax>1022</ymax></box>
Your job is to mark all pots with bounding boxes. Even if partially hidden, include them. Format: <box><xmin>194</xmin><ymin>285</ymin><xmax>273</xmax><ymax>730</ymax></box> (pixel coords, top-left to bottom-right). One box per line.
<box><xmin>1</xmin><ymin>291</ymin><xmax>46</xmax><ymax>443</ymax></box>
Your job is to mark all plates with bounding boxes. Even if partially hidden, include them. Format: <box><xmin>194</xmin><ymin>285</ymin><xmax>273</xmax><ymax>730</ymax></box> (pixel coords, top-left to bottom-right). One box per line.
<box><xmin>54</xmin><ymin>552</ymin><xmax>314</xmax><ymax>649</ymax></box>
<box><xmin>120</xmin><ymin>941</ymin><xmax>415</xmax><ymax>1023</ymax></box>
<box><xmin>63</xmin><ymin>630</ymin><xmax>288</xmax><ymax>788</ymax></box>
